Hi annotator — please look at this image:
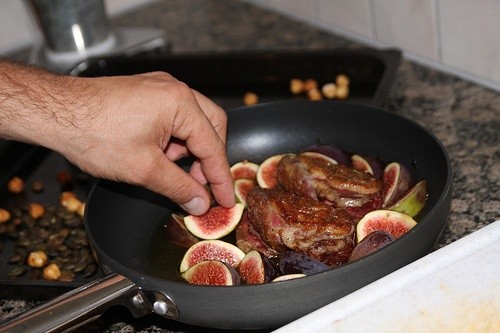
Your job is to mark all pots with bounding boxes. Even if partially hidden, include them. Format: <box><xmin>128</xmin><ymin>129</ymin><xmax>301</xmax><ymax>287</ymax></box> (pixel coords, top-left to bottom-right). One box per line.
<box><xmin>0</xmin><ymin>100</ymin><xmax>453</xmax><ymax>333</ymax></box>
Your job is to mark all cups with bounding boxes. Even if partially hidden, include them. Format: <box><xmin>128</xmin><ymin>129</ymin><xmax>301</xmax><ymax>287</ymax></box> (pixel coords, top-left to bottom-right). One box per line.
<box><xmin>26</xmin><ymin>0</ymin><xmax>118</xmax><ymax>66</ymax></box>
